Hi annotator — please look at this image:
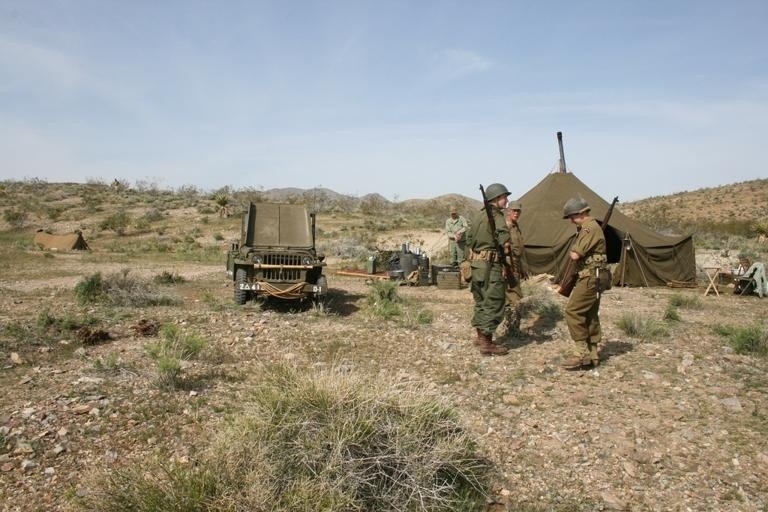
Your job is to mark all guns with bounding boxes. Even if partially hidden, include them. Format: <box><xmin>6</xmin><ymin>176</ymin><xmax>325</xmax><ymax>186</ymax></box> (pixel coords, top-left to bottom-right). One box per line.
<box><xmin>479</xmin><ymin>184</ymin><xmax>518</xmax><ymax>290</ymax></box>
<box><xmin>559</xmin><ymin>195</ymin><xmax>619</xmax><ymax>298</ymax></box>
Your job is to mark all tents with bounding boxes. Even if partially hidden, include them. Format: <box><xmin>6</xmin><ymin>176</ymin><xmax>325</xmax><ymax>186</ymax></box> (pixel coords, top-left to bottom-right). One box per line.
<box><xmin>502</xmin><ymin>171</ymin><xmax>698</xmax><ymax>289</ymax></box>
<box><xmin>33</xmin><ymin>231</ymin><xmax>88</xmax><ymax>251</ymax></box>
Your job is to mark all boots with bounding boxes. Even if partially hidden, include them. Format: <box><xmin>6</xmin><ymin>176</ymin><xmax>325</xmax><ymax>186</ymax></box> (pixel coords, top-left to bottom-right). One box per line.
<box><xmin>560</xmin><ymin>340</ymin><xmax>599</xmax><ymax>369</ymax></box>
<box><xmin>474</xmin><ymin>327</ymin><xmax>508</xmax><ymax>355</ymax></box>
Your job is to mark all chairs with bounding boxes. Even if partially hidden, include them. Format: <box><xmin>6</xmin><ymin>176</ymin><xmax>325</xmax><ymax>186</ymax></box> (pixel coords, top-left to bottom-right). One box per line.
<box><xmin>734</xmin><ymin>262</ymin><xmax>763</xmax><ymax>296</ymax></box>
<box><xmin>730</xmin><ymin>258</ymin><xmax>751</xmax><ymax>281</ymax></box>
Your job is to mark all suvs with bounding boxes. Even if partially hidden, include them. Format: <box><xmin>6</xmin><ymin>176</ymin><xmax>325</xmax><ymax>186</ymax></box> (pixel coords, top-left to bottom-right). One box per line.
<box><xmin>227</xmin><ymin>201</ymin><xmax>327</xmax><ymax>311</ymax></box>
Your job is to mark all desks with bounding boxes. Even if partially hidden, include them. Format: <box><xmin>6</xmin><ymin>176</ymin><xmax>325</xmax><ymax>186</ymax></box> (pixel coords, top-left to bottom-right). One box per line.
<box><xmin>697</xmin><ymin>265</ymin><xmax>721</xmax><ymax>297</ymax></box>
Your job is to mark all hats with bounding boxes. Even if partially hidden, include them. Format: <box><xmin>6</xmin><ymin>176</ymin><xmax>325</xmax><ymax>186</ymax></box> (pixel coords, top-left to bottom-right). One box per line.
<box><xmin>508</xmin><ymin>201</ymin><xmax>522</xmax><ymax>210</ymax></box>
<box><xmin>449</xmin><ymin>208</ymin><xmax>457</xmax><ymax>214</ymax></box>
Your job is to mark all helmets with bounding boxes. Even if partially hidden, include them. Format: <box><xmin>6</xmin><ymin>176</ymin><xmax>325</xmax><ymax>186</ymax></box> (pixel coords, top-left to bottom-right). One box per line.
<box><xmin>485</xmin><ymin>184</ymin><xmax>512</xmax><ymax>202</ymax></box>
<box><xmin>563</xmin><ymin>198</ymin><xmax>591</xmax><ymax>219</ymax></box>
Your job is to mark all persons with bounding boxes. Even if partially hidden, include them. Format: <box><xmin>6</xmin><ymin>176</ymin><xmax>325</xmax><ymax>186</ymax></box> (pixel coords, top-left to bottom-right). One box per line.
<box><xmin>466</xmin><ymin>183</ymin><xmax>512</xmax><ymax>356</ymax></box>
<box><xmin>560</xmin><ymin>198</ymin><xmax>608</xmax><ymax>369</ymax></box>
<box><xmin>446</xmin><ymin>206</ymin><xmax>469</xmax><ymax>285</ymax></box>
<box><xmin>505</xmin><ymin>201</ymin><xmax>531</xmax><ymax>338</ymax></box>
